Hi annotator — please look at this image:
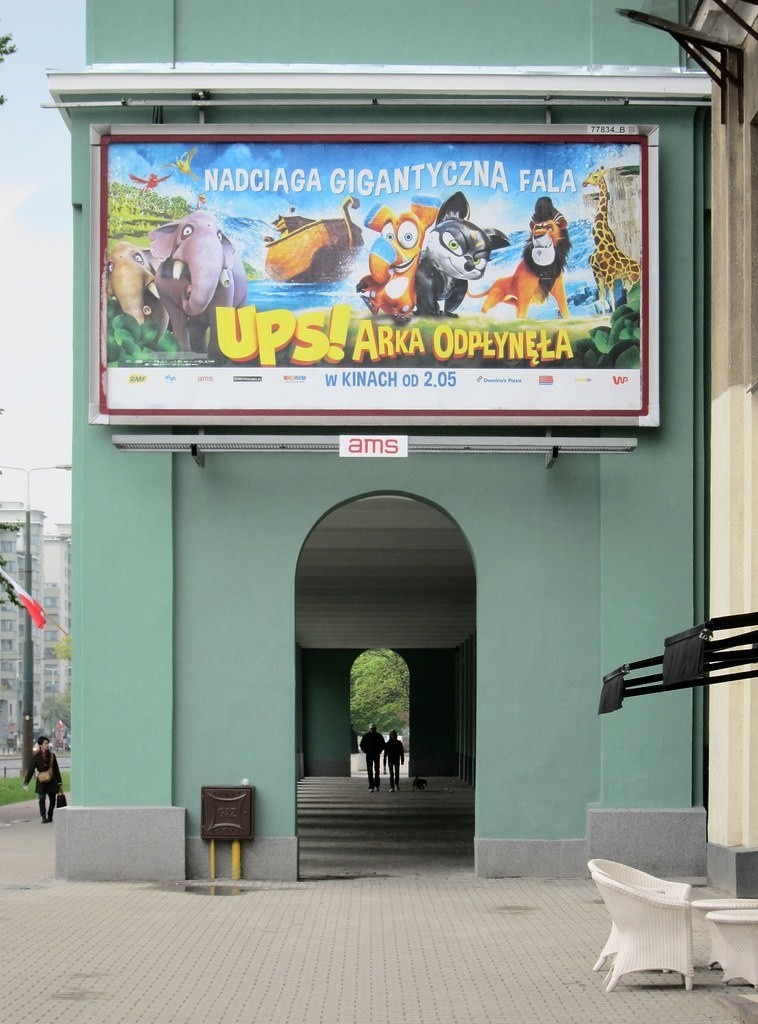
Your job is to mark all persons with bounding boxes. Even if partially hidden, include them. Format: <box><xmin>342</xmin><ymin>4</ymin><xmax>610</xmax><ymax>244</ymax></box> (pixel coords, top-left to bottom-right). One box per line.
<box><xmin>7</xmin><ymin>724</ymin><xmax>71</xmax><ymax>755</ymax></box>
<box><xmin>359</xmin><ymin>722</ymin><xmax>386</xmax><ymax>792</ymax></box>
<box><xmin>382</xmin><ymin>730</ymin><xmax>405</xmax><ymax>793</ymax></box>
<box><xmin>22</xmin><ymin>736</ymin><xmax>63</xmax><ymax>823</ymax></box>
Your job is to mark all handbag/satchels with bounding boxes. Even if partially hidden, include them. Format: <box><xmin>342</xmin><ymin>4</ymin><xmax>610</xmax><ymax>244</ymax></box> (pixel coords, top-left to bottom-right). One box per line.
<box><xmin>56</xmin><ymin>794</ymin><xmax>67</xmax><ymax>807</ymax></box>
<box><xmin>35</xmin><ymin>769</ymin><xmax>53</xmax><ymax>782</ymax></box>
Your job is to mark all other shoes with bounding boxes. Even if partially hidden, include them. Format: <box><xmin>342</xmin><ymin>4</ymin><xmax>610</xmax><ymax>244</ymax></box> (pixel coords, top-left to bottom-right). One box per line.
<box><xmin>41</xmin><ymin>809</ymin><xmax>53</xmax><ymax>823</ymax></box>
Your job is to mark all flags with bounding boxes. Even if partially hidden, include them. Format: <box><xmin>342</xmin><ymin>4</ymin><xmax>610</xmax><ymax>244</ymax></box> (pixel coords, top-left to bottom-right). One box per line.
<box><xmin>0</xmin><ymin>567</ymin><xmax>48</xmax><ymax>630</ymax></box>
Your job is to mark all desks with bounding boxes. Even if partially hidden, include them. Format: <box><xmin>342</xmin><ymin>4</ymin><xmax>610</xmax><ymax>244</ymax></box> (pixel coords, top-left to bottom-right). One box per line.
<box><xmin>705</xmin><ymin>909</ymin><xmax>757</xmax><ymax>989</ymax></box>
<box><xmin>688</xmin><ymin>898</ymin><xmax>757</xmax><ymax>968</ymax></box>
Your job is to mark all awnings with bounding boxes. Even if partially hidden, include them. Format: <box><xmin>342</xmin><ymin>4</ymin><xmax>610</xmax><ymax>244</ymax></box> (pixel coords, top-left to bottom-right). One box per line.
<box><xmin>596</xmin><ymin>637</ymin><xmax>757</xmax><ymax>717</ymax></box>
<box><xmin>656</xmin><ymin>609</ymin><xmax>757</xmax><ymax>690</ymax></box>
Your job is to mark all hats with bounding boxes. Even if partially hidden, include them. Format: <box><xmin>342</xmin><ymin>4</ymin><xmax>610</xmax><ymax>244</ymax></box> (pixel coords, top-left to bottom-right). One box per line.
<box><xmin>369</xmin><ymin>723</ymin><xmax>377</xmax><ymax>729</ymax></box>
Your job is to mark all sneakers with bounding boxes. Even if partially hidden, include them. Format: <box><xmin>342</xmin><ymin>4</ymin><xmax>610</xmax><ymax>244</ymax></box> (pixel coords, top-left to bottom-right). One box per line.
<box><xmin>389</xmin><ymin>784</ymin><xmax>400</xmax><ymax>792</ymax></box>
<box><xmin>369</xmin><ymin>786</ymin><xmax>380</xmax><ymax>793</ymax></box>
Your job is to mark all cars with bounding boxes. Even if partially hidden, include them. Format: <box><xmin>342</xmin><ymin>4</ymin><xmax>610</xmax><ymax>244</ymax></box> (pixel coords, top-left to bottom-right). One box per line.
<box><xmin>28</xmin><ymin>740</ymin><xmax>71</xmax><ymax>757</ymax></box>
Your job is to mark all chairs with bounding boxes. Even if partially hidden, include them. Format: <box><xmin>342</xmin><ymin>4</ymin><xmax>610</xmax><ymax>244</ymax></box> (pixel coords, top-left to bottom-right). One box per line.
<box><xmin>586</xmin><ymin>858</ymin><xmax>696</xmax><ymax>991</ymax></box>
